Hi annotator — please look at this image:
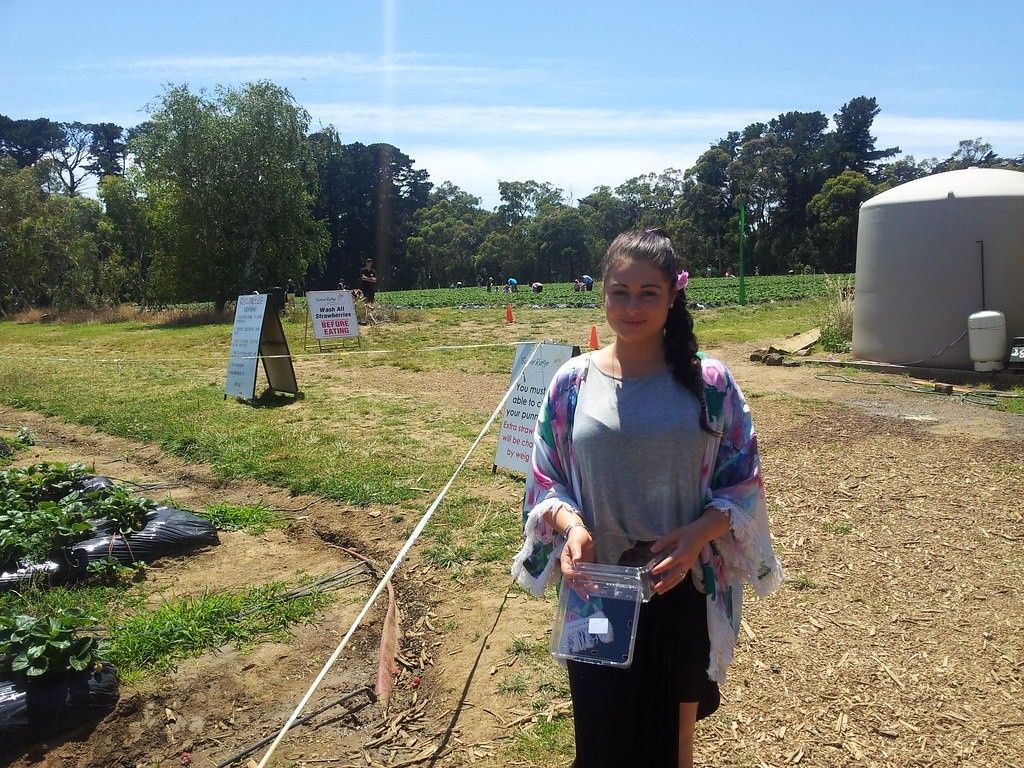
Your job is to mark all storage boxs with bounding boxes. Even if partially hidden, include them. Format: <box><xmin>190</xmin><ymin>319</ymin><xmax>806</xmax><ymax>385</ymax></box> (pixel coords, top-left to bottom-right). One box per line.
<box><xmin>548</xmin><ymin>535</ymin><xmax>677</xmax><ymax>670</ymax></box>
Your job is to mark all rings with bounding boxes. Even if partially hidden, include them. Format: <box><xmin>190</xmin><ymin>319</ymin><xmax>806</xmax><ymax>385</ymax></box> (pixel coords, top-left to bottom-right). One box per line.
<box><xmin>680</xmin><ymin>572</ymin><xmax>686</xmax><ymax>578</ymax></box>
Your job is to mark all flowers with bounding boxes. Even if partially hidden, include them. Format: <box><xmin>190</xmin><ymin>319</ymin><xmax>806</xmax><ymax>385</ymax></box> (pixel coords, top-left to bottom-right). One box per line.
<box><xmin>676</xmin><ymin>270</ymin><xmax>690</xmax><ymax>291</ymax></box>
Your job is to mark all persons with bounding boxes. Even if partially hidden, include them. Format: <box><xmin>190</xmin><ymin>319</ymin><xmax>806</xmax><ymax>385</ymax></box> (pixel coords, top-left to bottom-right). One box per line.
<box><xmin>573</xmin><ymin>279</ymin><xmax>581</xmax><ymax>291</ymax></box>
<box><xmin>358</xmin><ymin>258</ymin><xmax>377</xmax><ymax>304</ymax></box>
<box><xmin>754</xmin><ymin>266</ymin><xmax>760</xmax><ymax>276</ymax></box>
<box><xmin>725</xmin><ymin>265</ymin><xmax>732</xmax><ymax>276</ymax></box>
<box><xmin>285</xmin><ymin>278</ymin><xmax>297</xmax><ymax>309</ymax></box>
<box><xmin>449</xmin><ymin>283</ymin><xmax>455</xmax><ymax>289</ymax></box>
<box><xmin>494</xmin><ymin>287</ymin><xmax>501</xmax><ymax>293</ymax></box>
<box><xmin>580</xmin><ymin>274</ymin><xmax>593</xmax><ymax>291</ymax></box>
<box><xmin>456</xmin><ymin>282</ymin><xmax>462</xmax><ymax>289</ymax></box>
<box><xmin>486</xmin><ymin>277</ymin><xmax>493</xmax><ymax>292</ymax></box>
<box><xmin>706</xmin><ymin>266</ymin><xmax>712</xmax><ymax>278</ymax></box>
<box><xmin>476</xmin><ymin>275</ymin><xmax>482</xmax><ymax>287</ymax></box>
<box><xmin>529</xmin><ymin>282</ymin><xmax>543</xmax><ymax>293</ymax></box>
<box><xmin>510</xmin><ymin>228</ymin><xmax>785</xmax><ymax>768</ymax></box>
<box><xmin>337</xmin><ymin>279</ymin><xmax>346</xmax><ymax>290</ymax></box>
<box><xmin>507</xmin><ymin>278</ymin><xmax>517</xmax><ymax>292</ymax></box>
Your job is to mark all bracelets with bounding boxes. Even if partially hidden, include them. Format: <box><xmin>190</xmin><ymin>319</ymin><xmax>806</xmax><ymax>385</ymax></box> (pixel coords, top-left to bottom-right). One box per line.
<box><xmin>564</xmin><ymin>524</ymin><xmax>594</xmax><ymax>543</ymax></box>
